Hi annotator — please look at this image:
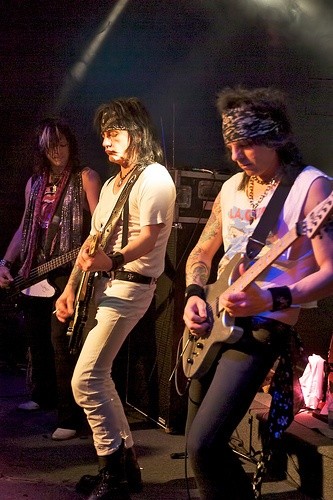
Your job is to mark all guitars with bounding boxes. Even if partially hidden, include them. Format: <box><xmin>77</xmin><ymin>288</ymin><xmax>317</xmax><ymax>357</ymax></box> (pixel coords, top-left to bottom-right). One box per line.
<box><xmin>182</xmin><ymin>190</ymin><xmax>333</xmax><ymax>380</ymax></box>
<box><xmin>66</xmin><ymin>231</ymin><xmax>101</xmax><ymax>354</ymax></box>
<box><xmin>0</xmin><ymin>247</ymin><xmax>82</xmax><ymax>320</ymax></box>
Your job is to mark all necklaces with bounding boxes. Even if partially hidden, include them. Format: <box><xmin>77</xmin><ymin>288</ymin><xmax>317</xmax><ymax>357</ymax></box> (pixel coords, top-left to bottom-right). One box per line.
<box><xmin>246</xmin><ymin>174</ymin><xmax>274</xmax><ymax>225</ymax></box>
<box><xmin>250</xmin><ymin>175</ymin><xmax>273</xmax><ymax>186</ymax></box>
<box><xmin>117</xmin><ymin>164</ymin><xmax>135</xmax><ymax>187</ymax></box>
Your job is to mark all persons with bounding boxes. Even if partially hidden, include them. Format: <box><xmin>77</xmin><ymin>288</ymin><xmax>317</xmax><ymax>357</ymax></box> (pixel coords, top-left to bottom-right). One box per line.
<box><xmin>186</xmin><ymin>83</ymin><xmax>333</xmax><ymax>500</ymax></box>
<box><xmin>0</xmin><ymin>121</ymin><xmax>102</xmax><ymax>440</ymax></box>
<box><xmin>54</xmin><ymin>96</ymin><xmax>176</xmax><ymax>500</ymax></box>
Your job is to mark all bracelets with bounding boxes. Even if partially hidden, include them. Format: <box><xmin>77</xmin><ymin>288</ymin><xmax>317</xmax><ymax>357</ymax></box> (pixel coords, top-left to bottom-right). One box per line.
<box><xmin>0</xmin><ymin>257</ymin><xmax>13</xmax><ymax>269</ymax></box>
<box><xmin>185</xmin><ymin>284</ymin><xmax>207</xmax><ymax>301</ymax></box>
<box><xmin>108</xmin><ymin>253</ymin><xmax>126</xmax><ymax>273</ymax></box>
<box><xmin>268</xmin><ymin>286</ymin><xmax>293</xmax><ymax>312</ymax></box>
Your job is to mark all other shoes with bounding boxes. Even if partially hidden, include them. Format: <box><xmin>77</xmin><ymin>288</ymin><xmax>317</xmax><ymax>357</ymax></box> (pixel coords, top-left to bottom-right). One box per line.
<box><xmin>51</xmin><ymin>428</ymin><xmax>76</xmax><ymax>440</ymax></box>
<box><xmin>89</xmin><ymin>468</ymin><xmax>130</xmax><ymax>500</ymax></box>
<box><xmin>78</xmin><ymin>462</ymin><xmax>142</xmax><ymax>491</ymax></box>
<box><xmin>18</xmin><ymin>400</ymin><xmax>40</xmax><ymax>409</ymax></box>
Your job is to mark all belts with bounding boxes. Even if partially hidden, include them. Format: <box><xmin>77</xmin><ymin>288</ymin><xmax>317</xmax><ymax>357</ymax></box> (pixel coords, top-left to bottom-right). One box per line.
<box><xmin>99</xmin><ymin>269</ymin><xmax>156</xmax><ymax>285</ymax></box>
